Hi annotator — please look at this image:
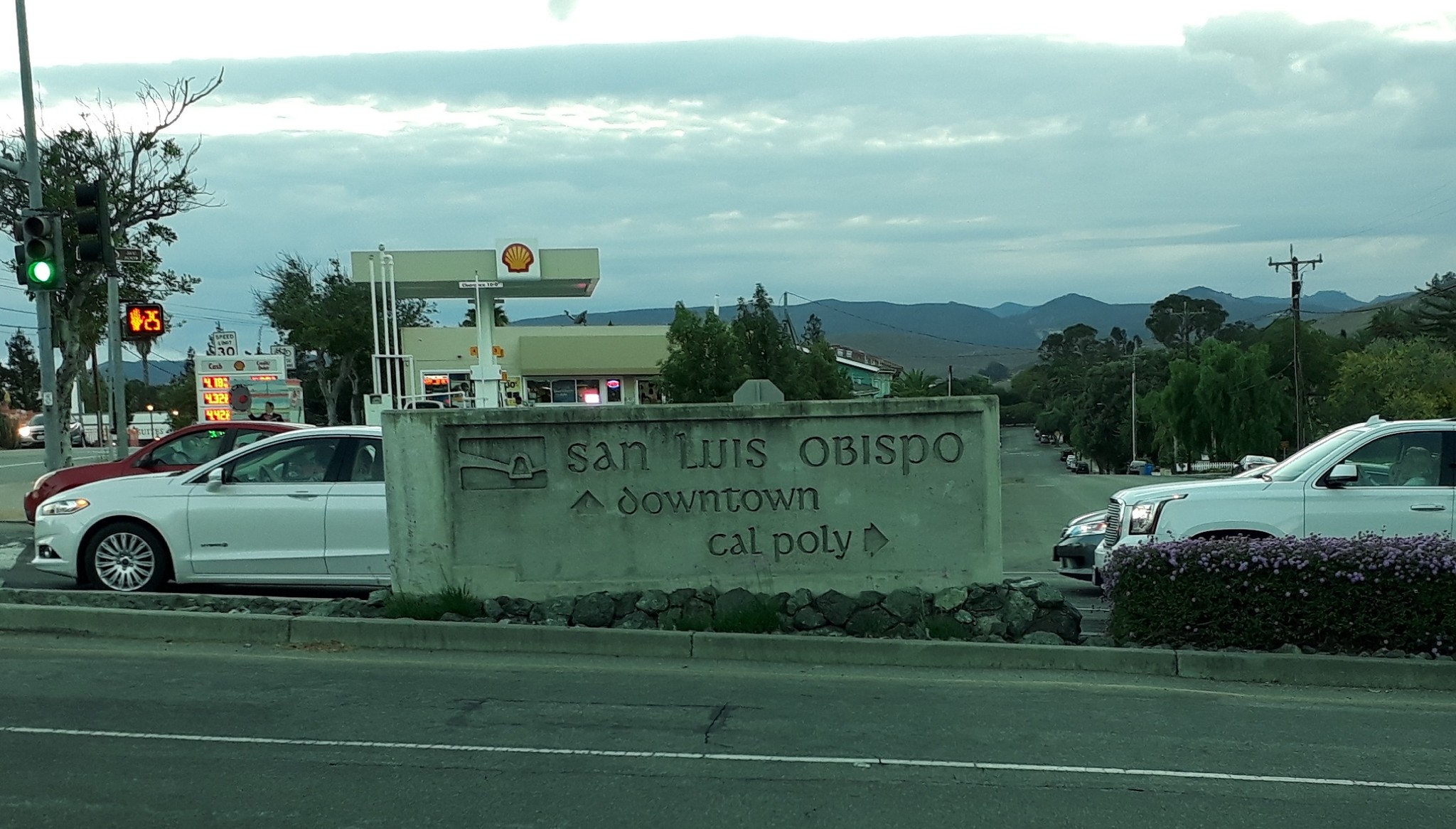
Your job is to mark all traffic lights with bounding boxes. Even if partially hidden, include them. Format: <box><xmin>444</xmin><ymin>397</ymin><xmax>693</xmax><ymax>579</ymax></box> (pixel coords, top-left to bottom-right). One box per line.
<box><xmin>75</xmin><ymin>173</ymin><xmax>115</xmax><ymax>266</ymax></box>
<box><xmin>11</xmin><ymin>218</ymin><xmax>29</xmax><ymax>285</ymax></box>
<box><xmin>21</xmin><ymin>212</ymin><xmax>66</xmax><ymax>294</ymax></box>
<box><xmin>126</xmin><ymin>304</ymin><xmax>166</xmax><ymax>337</ymax></box>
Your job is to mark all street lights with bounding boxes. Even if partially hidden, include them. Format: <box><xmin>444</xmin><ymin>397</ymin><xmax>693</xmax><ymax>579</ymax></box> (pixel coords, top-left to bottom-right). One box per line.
<box><xmin>146</xmin><ymin>405</ymin><xmax>155</xmax><ymax>440</ymax></box>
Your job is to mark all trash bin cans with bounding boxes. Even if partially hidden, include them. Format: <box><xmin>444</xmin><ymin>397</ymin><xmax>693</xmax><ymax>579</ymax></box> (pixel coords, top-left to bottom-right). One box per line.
<box><xmin>1144</xmin><ymin>464</ymin><xmax>1153</xmax><ymax>475</ymax></box>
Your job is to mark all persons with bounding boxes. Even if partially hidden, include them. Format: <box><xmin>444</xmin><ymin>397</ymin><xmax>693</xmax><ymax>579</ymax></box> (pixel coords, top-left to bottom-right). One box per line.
<box><xmin>245</xmin><ymin>401</ymin><xmax>285</xmax><ymax>423</ymax></box>
<box><xmin>203</xmin><ymin>437</ymin><xmax>222</xmax><ymax>464</ymax></box>
<box><xmin>309</xmin><ymin>447</ymin><xmax>334</xmax><ymax>482</ymax></box>
<box><xmin>1388</xmin><ymin>447</ymin><xmax>1431</xmax><ymax>487</ymax></box>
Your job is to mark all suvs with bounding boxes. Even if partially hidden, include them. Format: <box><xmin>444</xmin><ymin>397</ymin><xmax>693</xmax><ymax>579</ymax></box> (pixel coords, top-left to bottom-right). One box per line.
<box><xmin>1089</xmin><ymin>414</ymin><xmax>1456</xmax><ymax>596</ymax></box>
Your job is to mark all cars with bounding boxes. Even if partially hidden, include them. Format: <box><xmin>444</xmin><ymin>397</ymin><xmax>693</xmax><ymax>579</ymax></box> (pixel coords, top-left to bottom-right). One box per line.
<box><xmin>1033</xmin><ymin>426</ymin><xmax>1050</xmax><ymax>444</ymax></box>
<box><xmin>31</xmin><ymin>425</ymin><xmax>392</xmax><ymax>593</ymax></box>
<box><xmin>1231</xmin><ymin>455</ymin><xmax>1277</xmax><ymax>477</ymax></box>
<box><xmin>17</xmin><ymin>413</ymin><xmax>87</xmax><ymax>449</ymax></box>
<box><xmin>1060</xmin><ymin>449</ymin><xmax>1089</xmax><ymax>474</ymax></box>
<box><xmin>1051</xmin><ymin>460</ymin><xmax>1391</xmax><ymax>583</ymax></box>
<box><xmin>1127</xmin><ymin>460</ymin><xmax>1146</xmax><ymax>475</ymax></box>
<box><xmin>22</xmin><ymin>420</ymin><xmax>328</xmax><ymax>529</ymax></box>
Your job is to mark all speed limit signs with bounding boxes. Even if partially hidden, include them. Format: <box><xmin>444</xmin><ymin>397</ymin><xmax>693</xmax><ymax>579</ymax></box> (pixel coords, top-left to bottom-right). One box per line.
<box><xmin>213</xmin><ymin>331</ymin><xmax>237</xmax><ymax>358</ymax></box>
